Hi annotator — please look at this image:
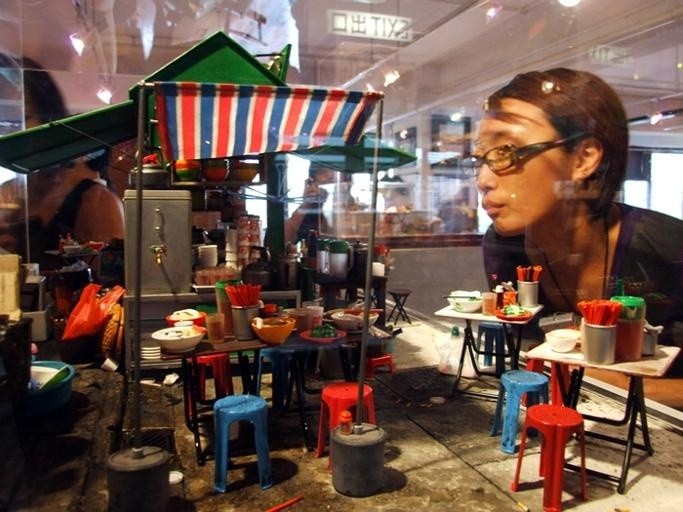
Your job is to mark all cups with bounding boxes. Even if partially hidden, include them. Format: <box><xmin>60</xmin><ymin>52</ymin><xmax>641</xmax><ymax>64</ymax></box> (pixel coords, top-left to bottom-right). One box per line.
<box><xmin>193</xmin><ymin>266</ymin><xmax>242</xmax><ymax>286</ymax></box>
<box><xmin>482</xmin><ymin>292</ymin><xmax>498</xmax><ymax>317</ymax></box>
<box><xmin>225</xmin><ymin>229</ymin><xmax>238</xmax><ymax>269</ymax></box>
<box><xmin>101</xmin><ymin>358</ymin><xmax>118</xmax><ymax>374</ymax></box>
<box><xmin>238</xmin><ymin>214</ymin><xmax>262</xmax><ymax>271</ymax></box>
<box><xmin>206</xmin><ymin>311</ymin><xmax>226</xmax><ymax>344</ymax></box>
<box><xmin>307</xmin><ymin>306</ymin><xmax>323</xmax><ymax>329</ymax></box>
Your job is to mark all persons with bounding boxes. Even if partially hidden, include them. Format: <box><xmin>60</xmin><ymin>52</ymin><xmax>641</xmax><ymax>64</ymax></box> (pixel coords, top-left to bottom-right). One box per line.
<box><xmin>474</xmin><ymin>68</ymin><xmax>683</xmax><ymax>380</ymax></box>
<box><xmin>0</xmin><ymin>53</ymin><xmax>125</xmax><ymax>262</ymax></box>
<box><xmin>289</xmin><ymin>159</ymin><xmax>482</xmax><ymax>241</ymax></box>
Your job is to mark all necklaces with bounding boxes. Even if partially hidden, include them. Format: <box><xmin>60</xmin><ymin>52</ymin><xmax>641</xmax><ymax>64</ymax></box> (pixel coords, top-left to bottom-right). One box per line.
<box><xmin>531</xmin><ymin>217</ymin><xmax>609</xmax><ymax>318</ymax></box>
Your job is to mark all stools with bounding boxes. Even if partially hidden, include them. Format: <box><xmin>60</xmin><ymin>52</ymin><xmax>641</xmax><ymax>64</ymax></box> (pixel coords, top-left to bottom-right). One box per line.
<box><xmin>387</xmin><ymin>289</ymin><xmax>412</xmax><ymax>325</ymax></box>
<box><xmin>489</xmin><ymin>369</ymin><xmax>549</xmax><ymax>454</ymax></box>
<box><xmin>187</xmin><ymin>353</ymin><xmax>234</xmax><ymax>416</ymax></box>
<box><xmin>521</xmin><ymin>343</ymin><xmax>570</xmax><ymax>407</ymax></box>
<box><xmin>213</xmin><ymin>394</ymin><xmax>272</xmax><ymax>492</ymax></box>
<box><xmin>364</xmin><ymin>353</ymin><xmax>396</xmax><ymax>377</ymax></box>
<box><xmin>257</xmin><ymin>349</ymin><xmax>306</xmax><ymax>407</ymax></box>
<box><xmin>512</xmin><ymin>404</ymin><xmax>590</xmax><ymax>512</ymax></box>
<box><xmin>315</xmin><ymin>382</ymin><xmax>377</xmax><ymax>474</ymax></box>
<box><xmin>475</xmin><ymin>322</ymin><xmax>519</xmax><ymax>379</ymax></box>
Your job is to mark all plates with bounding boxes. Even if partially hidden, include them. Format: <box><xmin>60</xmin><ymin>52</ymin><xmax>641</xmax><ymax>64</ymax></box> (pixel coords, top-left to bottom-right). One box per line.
<box><xmin>299</xmin><ymin>329</ymin><xmax>347</xmax><ymax>343</ymax></box>
<box><xmin>141</xmin><ymin>339</ymin><xmax>161</xmax><ymax>360</ymax></box>
<box><xmin>493</xmin><ymin>309</ymin><xmax>533</xmax><ymax>321</ymax></box>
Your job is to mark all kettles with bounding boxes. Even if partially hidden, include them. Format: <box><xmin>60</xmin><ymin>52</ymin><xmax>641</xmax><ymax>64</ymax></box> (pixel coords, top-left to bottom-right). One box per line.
<box><xmin>128</xmin><ymin>145</ymin><xmax>174</xmax><ymax>191</ymax></box>
<box><xmin>242</xmin><ymin>245</ymin><xmax>272</xmax><ymax>271</ymax></box>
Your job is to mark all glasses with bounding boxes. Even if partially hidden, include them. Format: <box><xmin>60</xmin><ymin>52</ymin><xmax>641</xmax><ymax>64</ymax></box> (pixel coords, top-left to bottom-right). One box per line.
<box><xmin>458</xmin><ymin>128</ymin><xmax>590</xmax><ymax>178</ymax></box>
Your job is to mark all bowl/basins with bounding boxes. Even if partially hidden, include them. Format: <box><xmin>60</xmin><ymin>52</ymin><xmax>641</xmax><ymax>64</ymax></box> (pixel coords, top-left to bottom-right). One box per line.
<box><xmin>175</xmin><ymin>158</ymin><xmax>260</xmax><ymax>182</ymax></box>
<box><xmin>89</xmin><ymin>241</ymin><xmax>106</xmax><ymax>252</ymax></box>
<box><xmin>251</xmin><ymin>316</ymin><xmax>298</xmax><ymax>345</ymax></box>
<box><xmin>63</xmin><ymin>246</ymin><xmax>83</xmax><ymax>255</ymax></box>
<box><xmin>446</xmin><ymin>296</ymin><xmax>482</xmax><ymax>313</ymax></box>
<box><xmin>29</xmin><ymin>359</ymin><xmax>76</xmax><ymax>413</ymax></box>
<box><xmin>151</xmin><ymin>327</ymin><xmax>204</xmax><ymax>354</ymax></box>
<box><xmin>198</xmin><ymin>244</ymin><xmax>218</xmax><ymax>267</ymax></box>
<box><xmin>330</xmin><ymin>308</ymin><xmax>380</xmax><ymax>335</ymax></box>
<box><xmin>164</xmin><ymin>311</ymin><xmax>207</xmax><ymax>327</ymax></box>
<box><xmin>544</xmin><ymin>329</ymin><xmax>581</xmax><ymax>354</ymax></box>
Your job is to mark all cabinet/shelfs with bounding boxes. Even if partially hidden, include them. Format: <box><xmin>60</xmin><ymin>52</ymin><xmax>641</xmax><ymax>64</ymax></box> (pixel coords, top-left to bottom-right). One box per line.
<box><xmin>123</xmin><ymin>290</ymin><xmax>301</xmax><ymax>382</ymax></box>
<box><xmin>171</xmin><ymin>154</ymin><xmax>268</xmax><ymax>185</ymax></box>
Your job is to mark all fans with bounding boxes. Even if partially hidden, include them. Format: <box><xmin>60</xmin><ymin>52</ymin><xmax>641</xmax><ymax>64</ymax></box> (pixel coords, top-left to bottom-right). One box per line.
<box><xmin>237</xmin><ymin>155</ymin><xmax>326</xmax><ymax>203</ymax></box>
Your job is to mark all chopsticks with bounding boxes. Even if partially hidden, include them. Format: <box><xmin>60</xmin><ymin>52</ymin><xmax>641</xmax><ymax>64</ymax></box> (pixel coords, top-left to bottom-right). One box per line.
<box><xmin>515</xmin><ymin>265</ymin><xmax>542</xmax><ymax>283</ymax></box>
<box><xmin>374</xmin><ymin>245</ymin><xmax>391</xmax><ymax>257</ymax></box>
<box><xmin>576</xmin><ymin>299</ymin><xmax>624</xmax><ymax>326</ymax></box>
<box><xmin>223</xmin><ymin>284</ymin><xmax>264</xmax><ymax>307</ymax></box>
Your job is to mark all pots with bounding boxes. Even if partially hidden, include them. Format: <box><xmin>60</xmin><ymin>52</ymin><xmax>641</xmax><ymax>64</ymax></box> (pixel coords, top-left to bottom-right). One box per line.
<box><xmin>350</xmin><ymin>239</ymin><xmax>369</xmax><ymax>265</ymax></box>
